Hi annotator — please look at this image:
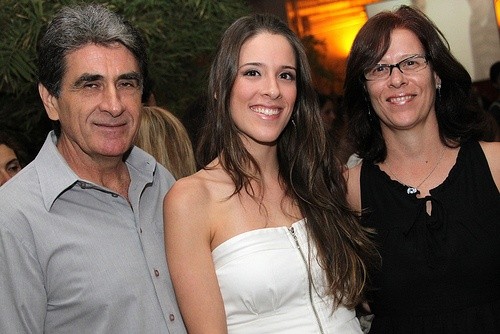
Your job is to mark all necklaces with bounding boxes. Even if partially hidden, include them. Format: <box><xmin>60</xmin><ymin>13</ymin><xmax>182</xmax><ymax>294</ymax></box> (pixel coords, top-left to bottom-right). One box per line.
<box><xmin>383</xmin><ymin>145</ymin><xmax>448</xmax><ymax>194</ymax></box>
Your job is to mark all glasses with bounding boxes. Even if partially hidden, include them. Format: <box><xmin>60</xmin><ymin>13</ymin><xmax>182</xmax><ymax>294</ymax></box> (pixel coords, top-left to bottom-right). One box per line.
<box><xmin>361</xmin><ymin>54</ymin><xmax>430</xmax><ymax>82</ymax></box>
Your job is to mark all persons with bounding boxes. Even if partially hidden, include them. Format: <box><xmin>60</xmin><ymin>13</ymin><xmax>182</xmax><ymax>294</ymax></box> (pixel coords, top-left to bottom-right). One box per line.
<box><xmin>161</xmin><ymin>12</ymin><xmax>387</xmax><ymax>334</ymax></box>
<box><xmin>136</xmin><ymin>104</ymin><xmax>199</xmax><ymax>181</ymax></box>
<box><xmin>1</xmin><ymin>0</ymin><xmax>188</xmax><ymax>334</ymax></box>
<box><xmin>0</xmin><ymin>135</ymin><xmax>30</xmax><ymax>186</ymax></box>
<box><xmin>141</xmin><ymin>89</ymin><xmax>157</xmax><ymax>106</ymax></box>
<box><xmin>322</xmin><ymin>5</ymin><xmax>500</xmax><ymax>334</ymax></box>
<box><xmin>311</xmin><ymin>59</ymin><xmax>500</xmax><ymax>177</ymax></box>
